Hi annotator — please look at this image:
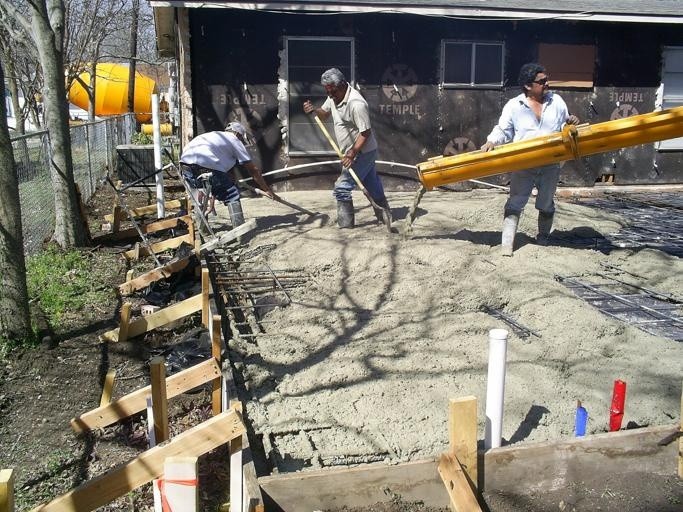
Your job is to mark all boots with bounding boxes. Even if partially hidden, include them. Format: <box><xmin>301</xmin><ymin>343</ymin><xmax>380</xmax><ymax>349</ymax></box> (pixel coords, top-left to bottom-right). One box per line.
<box><xmin>198</xmin><ymin>204</ymin><xmax>208</xmax><ymax>229</ymax></box>
<box><xmin>536</xmin><ymin>211</ymin><xmax>554</xmax><ymax>245</ymax></box>
<box><xmin>371</xmin><ymin>198</ymin><xmax>392</xmax><ymax>226</ymax></box>
<box><xmin>227</xmin><ymin>201</ymin><xmax>255</xmax><ymax>245</ymax></box>
<box><xmin>336</xmin><ymin>199</ymin><xmax>354</xmax><ymax>229</ymax></box>
<box><xmin>501</xmin><ymin>214</ymin><xmax>519</xmax><ymax>256</ymax></box>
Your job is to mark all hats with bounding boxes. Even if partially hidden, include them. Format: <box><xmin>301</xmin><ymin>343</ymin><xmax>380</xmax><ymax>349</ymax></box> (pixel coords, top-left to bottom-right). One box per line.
<box><xmin>223</xmin><ymin>121</ymin><xmax>248</xmax><ymax>145</ymax></box>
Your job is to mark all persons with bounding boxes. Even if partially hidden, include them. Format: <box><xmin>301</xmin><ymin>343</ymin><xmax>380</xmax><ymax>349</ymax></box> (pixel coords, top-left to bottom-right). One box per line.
<box><xmin>179</xmin><ymin>121</ymin><xmax>281</xmax><ymax>246</ymax></box>
<box><xmin>481</xmin><ymin>62</ymin><xmax>581</xmax><ymax>256</ymax></box>
<box><xmin>303</xmin><ymin>67</ymin><xmax>388</xmax><ymax>227</ymax></box>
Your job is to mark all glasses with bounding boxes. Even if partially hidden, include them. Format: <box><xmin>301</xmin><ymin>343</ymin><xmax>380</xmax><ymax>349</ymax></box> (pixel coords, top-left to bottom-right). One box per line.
<box><xmin>531</xmin><ymin>77</ymin><xmax>547</xmax><ymax>85</ymax></box>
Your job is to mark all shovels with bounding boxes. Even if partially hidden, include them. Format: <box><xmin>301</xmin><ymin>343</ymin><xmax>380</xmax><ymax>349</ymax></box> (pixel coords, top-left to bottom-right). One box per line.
<box><xmin>304</xmin><ymin>103</ymin><xmax>392</xmax><ymax>234</ymax></box>
